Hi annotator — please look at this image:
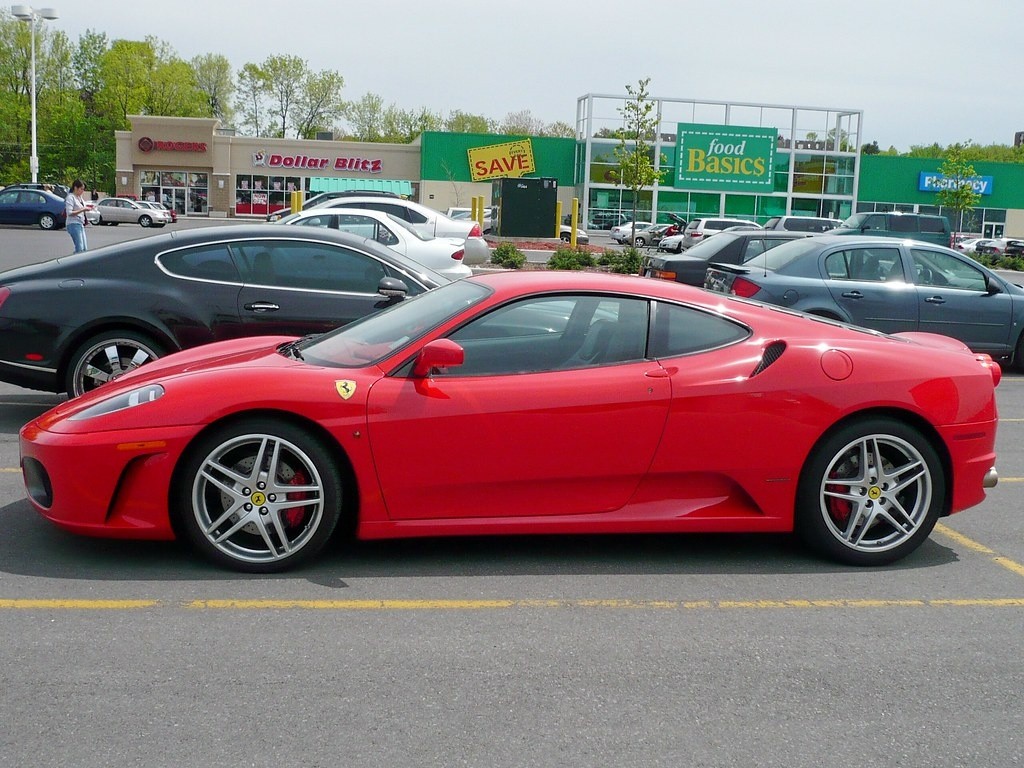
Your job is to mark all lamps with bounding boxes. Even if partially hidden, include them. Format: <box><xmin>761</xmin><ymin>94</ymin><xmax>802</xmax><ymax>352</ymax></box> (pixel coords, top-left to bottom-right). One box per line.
<box><xmin>121</xmin><ymin>176</ymin><xmax>128</xmax><ymax>186</ymax></box>
<box><xmin>218</xmin><ymin>180</ymin><xmax>225</xmax><ymax>189</ymax></box>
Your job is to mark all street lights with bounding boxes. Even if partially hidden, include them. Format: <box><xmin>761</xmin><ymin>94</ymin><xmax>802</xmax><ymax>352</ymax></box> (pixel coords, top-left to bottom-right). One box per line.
<box><xmin>11</xmin><ymin>4</ymin><xmax>61</xmax><ymax>183</ymax></box>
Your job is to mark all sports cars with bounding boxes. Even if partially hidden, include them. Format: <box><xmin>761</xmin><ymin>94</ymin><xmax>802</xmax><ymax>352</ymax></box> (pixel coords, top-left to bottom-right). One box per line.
<box><xmin>19</xmin><ymin>269</ymin><xmax>1001</xmax><ymax>576</ymax></box>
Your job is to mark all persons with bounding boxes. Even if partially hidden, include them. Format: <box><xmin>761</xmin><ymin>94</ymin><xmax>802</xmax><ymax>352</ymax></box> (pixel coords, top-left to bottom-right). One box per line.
<box><xmin>66</xmin><ymin>180</ymin><xmax>93</xmax><ymax>253</ymax></box>
<box><xmin>91</xmin><ymin>188</ymin><xmax>99</xmax><ymax>200</ymax></box>
<box><xmin>44</xmin><ymin>184</ymin><xmax>53</xmax><ymax>194</ymax></box>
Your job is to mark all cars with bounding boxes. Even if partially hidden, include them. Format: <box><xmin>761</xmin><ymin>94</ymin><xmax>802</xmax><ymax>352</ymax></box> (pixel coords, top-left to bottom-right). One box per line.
<box><xmin>295</xmin><ymin>196</ymin><xmax>490</xmax><ymax>265</ymax></box>
<box><xmin>0</xmin><ymin>225</ymin><xmax>617</xmax><ymax>400</ymax></box>
<box><xmin>610</xmin><ymin>211</ymin><xmax>1024</xmax><ymax>260</ymax></box>
<box><xmin>266</xmin><ymin>189</ymin><xmax>400</xmax><ymax>222</ymax></box>
<box><xmin>182</xmin><ymin>208</ymin><xmax>473</xmax><ymax>290</ymax></box>
<box><xmin>446</xmin><ymin>206</ymin><xmax>589</xmax><ymax>244</ymax></box>
<box><xmin>0</xmin><ymin>184</ymin><xmax>172</xmax><ymax>229</ymax></box>
<box><xmin>705</xmin><ymin>236</ymin><xmax>1024</xmax><ymax>376</ymax></box>
<box><xmin>638</xmin><ymin>231</ymin><xmax>957</xmax><ymax>287</ymax></box>
<box><xmin>491</xmin><ymin>205</ymin><xmax>501</xmax><ymax>236</ymax></box>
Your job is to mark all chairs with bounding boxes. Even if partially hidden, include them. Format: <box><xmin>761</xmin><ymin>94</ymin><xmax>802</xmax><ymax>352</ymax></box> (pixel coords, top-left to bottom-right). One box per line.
<box><xmin>564</xmin><ymin>319</ymin><xmax>616</xmax><ymax>366</ymax></box>
<box><xmin>888</xmin><ymin>257</ymin><xmax>902</xmax><ymax>281</ymax></box>
<box><xmin>607</xmin><ymin>303</ymin><xmax>646</xmax><ymax>362</ymax></box>
<box><xmin>250</xmin><ymin>252</ymin><xmax>283</xmax><ymax>286</ymax></box>
<box><xmin>860</xmin><ymin>256</ymin><xmax>882</xmax><ymax>279</ymax></box>
